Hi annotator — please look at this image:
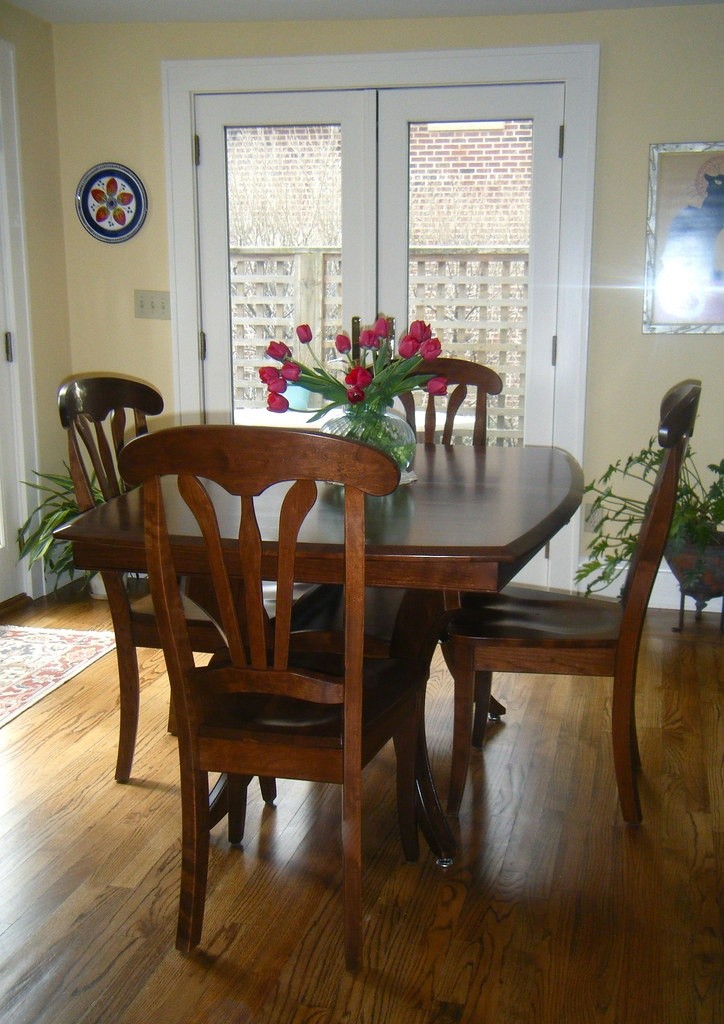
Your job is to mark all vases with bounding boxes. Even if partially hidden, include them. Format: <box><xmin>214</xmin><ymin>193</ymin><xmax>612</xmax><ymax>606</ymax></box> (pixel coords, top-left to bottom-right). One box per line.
<box><xmin>317</xmin><ymin>409</ymin><xmax>417</xmax><ymax>486</ymax></box>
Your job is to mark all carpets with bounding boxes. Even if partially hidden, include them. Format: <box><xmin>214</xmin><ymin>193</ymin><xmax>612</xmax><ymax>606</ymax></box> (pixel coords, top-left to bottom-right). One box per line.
<box><xmin>0</xmin><ymin>625</ymin><xmax>117</xmax><ymax>729</ymax></box>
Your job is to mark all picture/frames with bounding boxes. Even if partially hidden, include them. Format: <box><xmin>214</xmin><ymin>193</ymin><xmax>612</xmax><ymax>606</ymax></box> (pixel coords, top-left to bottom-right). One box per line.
<box><xmin>643</xmin><ymin>141</ymin><xmax>724</xmax><ymax>335</ymax></box>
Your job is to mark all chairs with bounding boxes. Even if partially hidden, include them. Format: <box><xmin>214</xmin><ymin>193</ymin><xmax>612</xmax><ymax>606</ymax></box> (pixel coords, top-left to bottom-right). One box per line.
<box><xmin>58</xmin><ymin>377</ymin><xmax>338</xmax><ymax>805</ymax></box>
<box><xmin>442</xmin><ymin>378</ymin><xmax>703</xmax><ymax>827</ymax></box>
<box><xmin>117</xmin><ymin>424</ymin><xmax>432</xmax><ymax>974</ymax></box>
<box><xmin>365</xmin><ymin>352</ymin><xmax>506</xmax><ymax>719</ymax></box>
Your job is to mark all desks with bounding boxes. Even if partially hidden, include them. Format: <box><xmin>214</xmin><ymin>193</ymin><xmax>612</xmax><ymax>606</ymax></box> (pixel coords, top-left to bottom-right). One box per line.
<box><xmin>51</xmin><ymin>443</ymin><xmax>585</xmax><ymax>868</ymax></box>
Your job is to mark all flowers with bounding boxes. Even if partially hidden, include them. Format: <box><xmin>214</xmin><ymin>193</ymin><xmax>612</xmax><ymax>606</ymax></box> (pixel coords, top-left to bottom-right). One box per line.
<box><xmin>258</xmin><ymin>318</ymin><xmax>449</xmax><ymax>464</ymax></box>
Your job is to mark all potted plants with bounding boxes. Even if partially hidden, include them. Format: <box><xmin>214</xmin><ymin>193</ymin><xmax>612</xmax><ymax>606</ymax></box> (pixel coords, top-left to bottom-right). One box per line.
<box><xmin>14</xmin><ymin>459</ymin><xmax>141</xmax><ymax>600</ymax></box>
<box><xmin>572</xmin><ymin>427</ymin><xmax>724</xmax><ymax>612</ymax></box>
